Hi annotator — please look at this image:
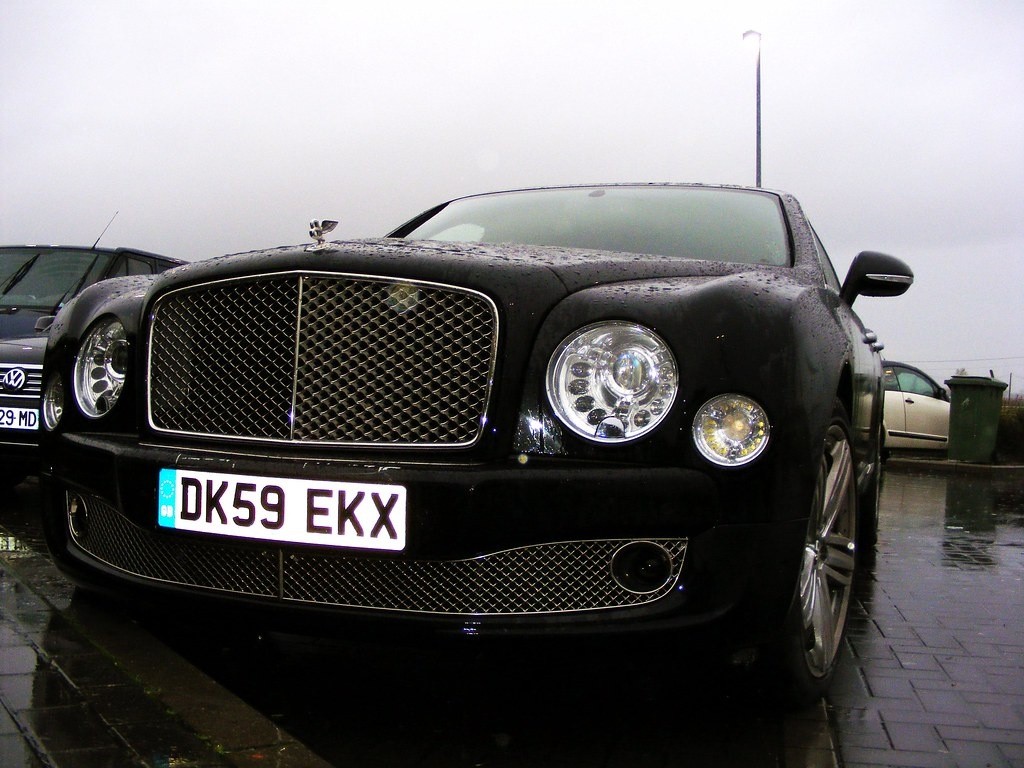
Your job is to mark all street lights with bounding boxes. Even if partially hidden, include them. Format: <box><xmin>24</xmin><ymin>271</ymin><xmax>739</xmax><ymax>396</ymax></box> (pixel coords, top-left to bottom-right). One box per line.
<box><xmin>745</xmin><ymin>28</ymin><xmax>764</xmax><ymax>185</ymax></box>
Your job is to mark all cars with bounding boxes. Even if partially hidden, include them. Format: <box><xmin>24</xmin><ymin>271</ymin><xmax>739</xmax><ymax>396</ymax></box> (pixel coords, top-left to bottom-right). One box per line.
<box><xmin>882</xmin><ymin>360</ymin><xmax>953</xmax><ymax>463</ymax></box>
<box><xmin>0</xmin><ymin>243</ymin><xmax>191</xmax><ymax>490</ymax></box>
<box><xmin>38</xmin><ymin>180</ymin><xmax>918</xmax><ymax>722</ymax></box>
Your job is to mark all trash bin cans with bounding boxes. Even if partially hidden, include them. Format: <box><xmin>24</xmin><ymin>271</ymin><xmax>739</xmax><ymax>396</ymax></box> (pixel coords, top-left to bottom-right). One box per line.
<box><xmin>944</xmin><ymin>375</ymin><xmax>1009</xmax><ymax>463</ymax></box>
